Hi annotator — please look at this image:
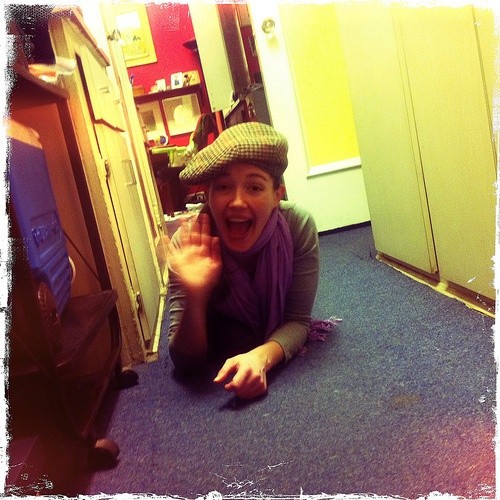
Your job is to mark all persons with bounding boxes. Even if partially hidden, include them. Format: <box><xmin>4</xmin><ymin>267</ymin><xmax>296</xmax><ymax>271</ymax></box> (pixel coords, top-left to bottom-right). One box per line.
<box><xmin>161</xmin><ymin>122</ymin><xmax>320</xmax><ymax>398</ymax></box>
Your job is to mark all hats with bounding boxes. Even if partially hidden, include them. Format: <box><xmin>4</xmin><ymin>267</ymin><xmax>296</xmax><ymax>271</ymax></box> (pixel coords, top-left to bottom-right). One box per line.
<box><xmin>179</xmin><ymin>122</ymin><xmax>288</xmax><ymax>191</ymax></box>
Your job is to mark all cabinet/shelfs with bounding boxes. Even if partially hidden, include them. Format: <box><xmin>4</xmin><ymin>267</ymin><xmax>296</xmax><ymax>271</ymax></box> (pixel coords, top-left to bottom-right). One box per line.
<box><xmin>51</xmin><ymin>13</ymin><xmax>167</xmax><ymax>396</ymax></box>
